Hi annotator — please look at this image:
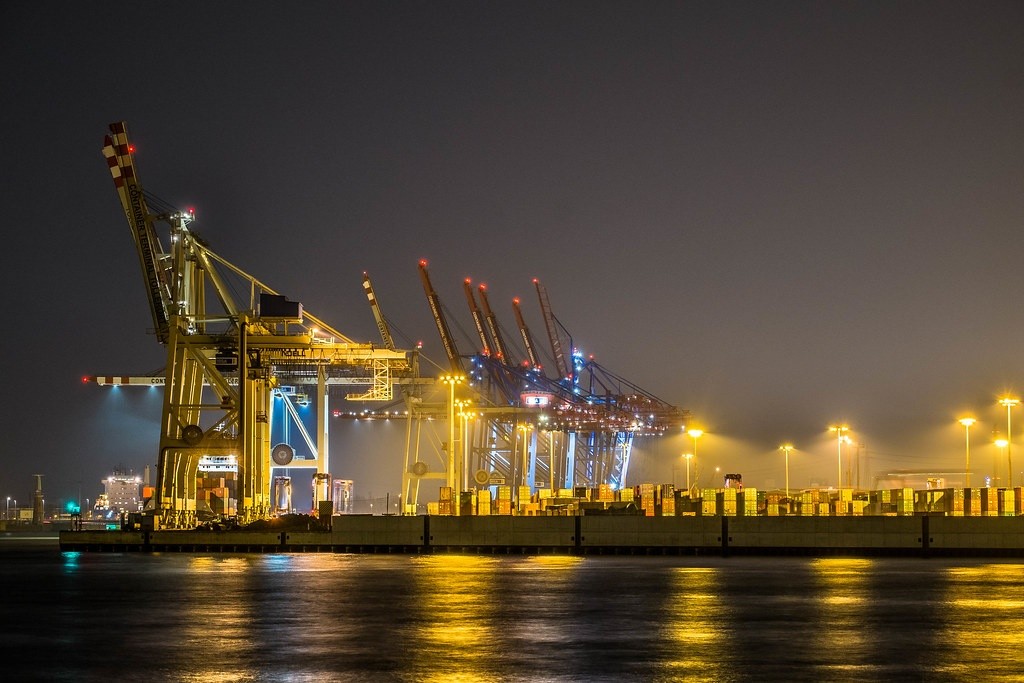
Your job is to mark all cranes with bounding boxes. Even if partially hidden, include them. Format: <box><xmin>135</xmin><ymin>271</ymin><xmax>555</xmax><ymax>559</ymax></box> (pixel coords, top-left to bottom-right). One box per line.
<box><xmin>76</xmin><ymin>115</ymin><xmax>693</xmax><ymax>514</ymax></box>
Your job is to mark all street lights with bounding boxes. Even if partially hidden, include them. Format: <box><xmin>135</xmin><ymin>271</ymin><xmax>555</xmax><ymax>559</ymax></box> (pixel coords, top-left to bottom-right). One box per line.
<box><xmin>837</xmin><ymin>434</ymin><xmax>855</xmax><ymax>488</ymax></box>
<box><xmin>828</xmin><ymin>424</ymin><xmax>851</xmax><ymax>487</ymax></box>
<box><xmin>778</xmin><ymin>443</ymin><xmax>793</xmax><ymax>498</ymax></box>
<box><xmin>997</xmin><ymin>397</ymin><xmax>1020</xmax><ymax>486</ymax></box>
<box><xmin>995</xmin><ymin>438</ymin><xmax>1010</xmax><ymax>488</ymax></box>
<box><xmin>682</xmin><ymin>453</ymin><xmax>693</xmax><ymax>493</ymax></box>
<box><xmin>687</xmin><ymin>429</ymin><xmax>703</xmax><ymax>489</ymax></box>
<box><xmin>959</xmin><ymin>416</ymin><xmax>975</xmax><ymax>491</ymax></box>
